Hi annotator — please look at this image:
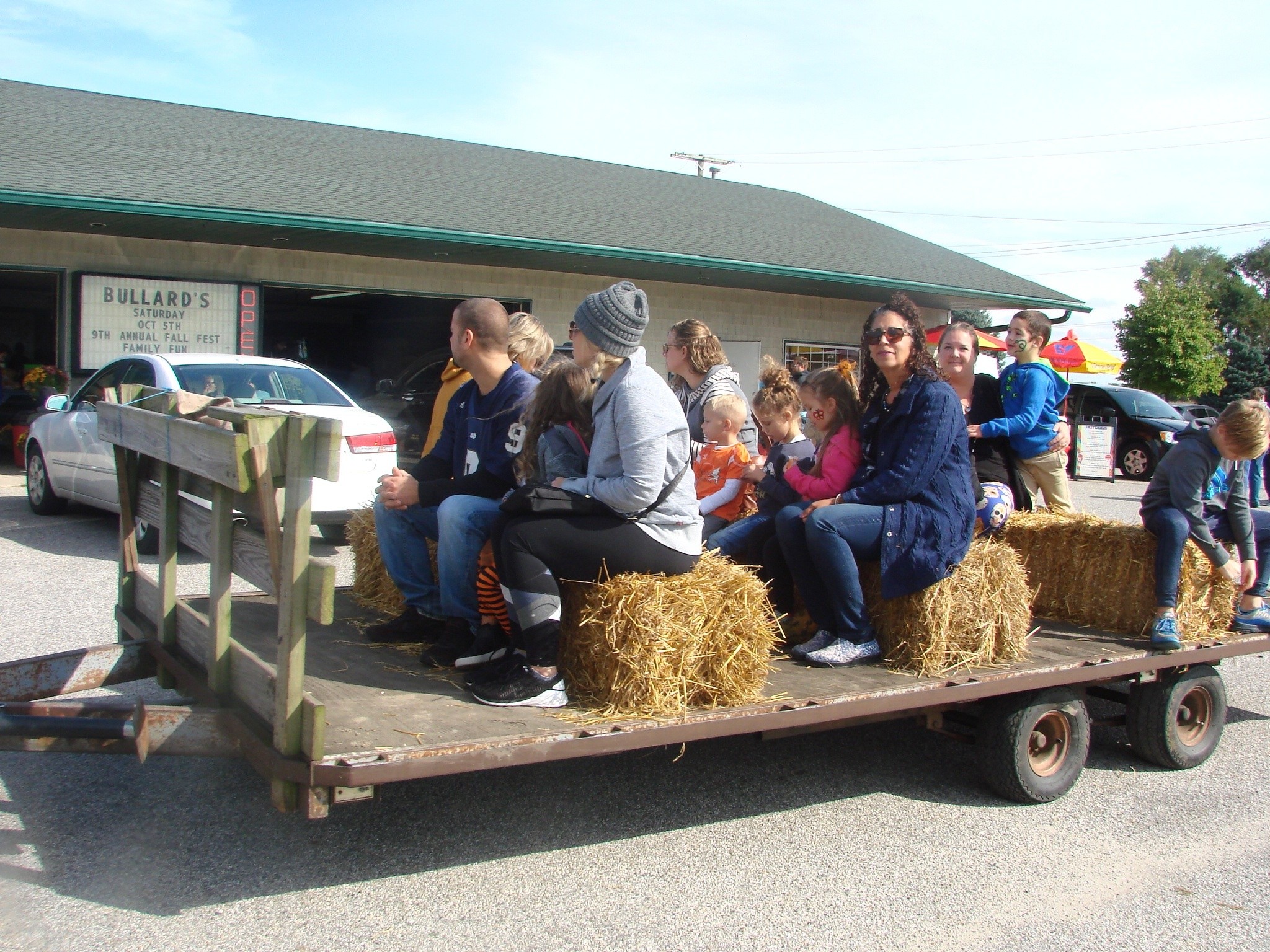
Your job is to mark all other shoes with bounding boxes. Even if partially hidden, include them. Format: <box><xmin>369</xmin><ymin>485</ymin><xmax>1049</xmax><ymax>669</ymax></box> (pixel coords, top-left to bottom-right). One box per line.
<box><xmin>806</xmin><ymin>637</ymin><xmax>883</xmax><ymax>668</ymax></box>
<box><xmin>790</xmin><ymin>631</ymin><xmax>839</xmax><ymax>663</ymax></box>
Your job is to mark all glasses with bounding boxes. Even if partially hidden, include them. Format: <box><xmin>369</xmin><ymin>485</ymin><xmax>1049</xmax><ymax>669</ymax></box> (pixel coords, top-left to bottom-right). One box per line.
<box><xmin>864</xmin><ymin>327</ymin><xmax>912</xmax><ymax>345</ymax></box>
<box><xmin>662</xmin><ymin>343</ymin><xmax>677</xmax><ymax>352</ymax></box>
<box><xmin>569</xmin><ymin>321</ymin><xmax>580</xmax><ymax>340</ymax></box>
<box><xmin>791</xmin><ymin>363</ymin><xmax>799</xmax><ymax>367</ymax></box>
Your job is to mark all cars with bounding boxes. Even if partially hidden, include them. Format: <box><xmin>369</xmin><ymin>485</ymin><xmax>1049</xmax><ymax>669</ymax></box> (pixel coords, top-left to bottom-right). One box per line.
<box><xmin>20</xmin><ymin>350</ymin><xmax>398</xmax><ymax>555</ymax></box>
<box><xmin>354</xmin><ymin>347</ymin><xmax>572</xmax><ymax>467</ymax></box>
<box><xmin>1173</xmin><ymin>403</ymin><xmax>1218</xmax><ymax>423</ymax></box>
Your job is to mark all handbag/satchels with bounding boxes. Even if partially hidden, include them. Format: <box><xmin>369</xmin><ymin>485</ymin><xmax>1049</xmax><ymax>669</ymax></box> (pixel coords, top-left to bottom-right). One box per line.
<box><xmin>499</xmin><ymin>482</ymin><xmax>626</xmax><ymax>521</ymax></box>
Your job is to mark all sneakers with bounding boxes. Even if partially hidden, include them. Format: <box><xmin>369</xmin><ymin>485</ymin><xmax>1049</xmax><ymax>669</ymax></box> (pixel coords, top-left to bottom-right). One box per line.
<box><xmin>462</xmin><ymin>654</ymin><xmax>526</xmax><ymax>686</ymax></box>
<box><xmin>420</xmin><ymin>617</ymin><xmax>474</xmax><ymax>667</ymax></box>
<box><xmin>1150</xmin><ymin>611</ymin><xmax>1181</xmax><ymax>651</ymax></box>
<box><xmin>471</xmin><ymin>663</ymin><xmax>569</xmax><ymax>707</ymax></box>
<box><xmin>454</xmin><ymin>624</ymin><xmax>508</xmax><ymax>666</ymax></box>
<box><xmin>365</xmin><ymin>611</ymin><xmax>444</xmax><ymax>644</ymax></box>
<box><xmin>1231</xmin><ymin>601</ymin><xmax>1270</xmax><ymax>632</ymax></box>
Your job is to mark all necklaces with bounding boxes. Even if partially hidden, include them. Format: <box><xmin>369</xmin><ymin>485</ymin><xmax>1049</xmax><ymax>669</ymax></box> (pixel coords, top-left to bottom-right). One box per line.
<box><xmin>961</xmin><ymin>394</ymin><xmax>972</xmax><ymax>412</ymax></box>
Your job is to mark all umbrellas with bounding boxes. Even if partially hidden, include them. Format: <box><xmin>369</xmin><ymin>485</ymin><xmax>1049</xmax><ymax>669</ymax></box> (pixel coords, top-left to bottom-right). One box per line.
<box><xmin>923</xmin><ymin>318</ymin><xmax>1007</xmax><ymax>350</ymax></box>
<box><xmin>1042</xmin><ymin>326</ymin><xmax>1125</xmax><ymax>416</ymax></box>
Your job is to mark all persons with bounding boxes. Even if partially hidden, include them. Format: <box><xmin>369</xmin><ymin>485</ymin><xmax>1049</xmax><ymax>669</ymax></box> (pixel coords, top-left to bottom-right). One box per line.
<box><xmin>966</xmin><ymin>310</ymin><xmax>1075</xmax><ymax>514</ymax></box>
<box><xmin>420</xmin><ymin>312</ymin><xmax>555</xmax><ymax>458</ymax></box>
<box><xmin>462</xmin><ymin>280</ymin><xmax>704</xmax><ymax>706</ymax></box>
<box><xmin>745</xmin><ymin>360</ymin><xmax>861</xmax><ymax>624</ymax></box>
<box><xmin>1141</xmin><ymin>399</ymin><xmax>1270</xmax><ymax>650</ymax></box>
<box><xmin>774</xmin><ymin>292</ymin><xmax>976</xmax><ymax>666</ymax></box>
<box><xmin>1262</xmin><ymin>391</ymin><xmax>1270</xmax><ymax>506</ymax></box>
<box><xmin>364</xmin><ymin>297</ymin><xmax>542</xmax><ymax>666</ymax></box>
<box><xmin>454</xmin><ymin>362</ymin><xmax>594</xmax><ymax>669</ymax></box>
<box><xmin>789</xmin><ymin>357</ymin><xmax>811</xmax><ymax>386</ymax></box>
<box><xmin>707</xmin><ymin>368</ymin><xmax>817</xmax><ymax>554</ymax></box>
<box><xmin>663</xmin><ymin>319</ymin><xmax>759</xmax><ymax>463</ymax></box>
<box><xmin>1243</xmin><ymin>386</ymin><xmax>1265</xmax><ymax>509</ymax></box>
<box><xmin>935</xmin><ymin>323</ymin><xmax>1071</xmax><ymax>511</ymax></box>
<box><xmin>199</xmin><ymin>374</ymin><xmax>257</xmax><ymax>398</ymax></box>
<box><xmin>693</xmin><ymin>394</ymin><xmax>751</xmax><ymax>546</ymax></box>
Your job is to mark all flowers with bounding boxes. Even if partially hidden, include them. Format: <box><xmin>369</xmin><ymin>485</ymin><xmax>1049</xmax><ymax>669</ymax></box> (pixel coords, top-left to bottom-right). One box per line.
<box><xmin>23</xmin><ymin>367</ymin><xmax>69</xmax><ymax>406</ymax></box>
<box><xmin>16</xmin><ymin>430</ymin><xmax>29</xmax><ymax>453</ymax></box>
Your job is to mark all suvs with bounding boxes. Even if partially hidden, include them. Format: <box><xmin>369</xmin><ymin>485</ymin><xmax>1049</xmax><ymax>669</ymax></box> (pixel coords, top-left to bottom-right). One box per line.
<box><xmin>1065</xmin><ymin>381</ymin><xmax>1193</xmax><ymax>481</ymax></box>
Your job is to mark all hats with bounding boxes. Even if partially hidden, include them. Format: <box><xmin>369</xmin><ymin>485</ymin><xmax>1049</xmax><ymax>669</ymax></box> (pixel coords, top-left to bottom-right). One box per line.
<box><xmin>573</xmin><ymin>279</ymin><xmax>649</xmax><ymax>357</ymax></box>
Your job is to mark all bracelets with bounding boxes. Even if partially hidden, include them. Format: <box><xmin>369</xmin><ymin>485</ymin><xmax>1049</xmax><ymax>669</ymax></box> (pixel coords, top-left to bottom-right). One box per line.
<box><xmin>835</xmin><ymin>493</ymin><xmax>841</xmax><ymax>504</ymax></box>
